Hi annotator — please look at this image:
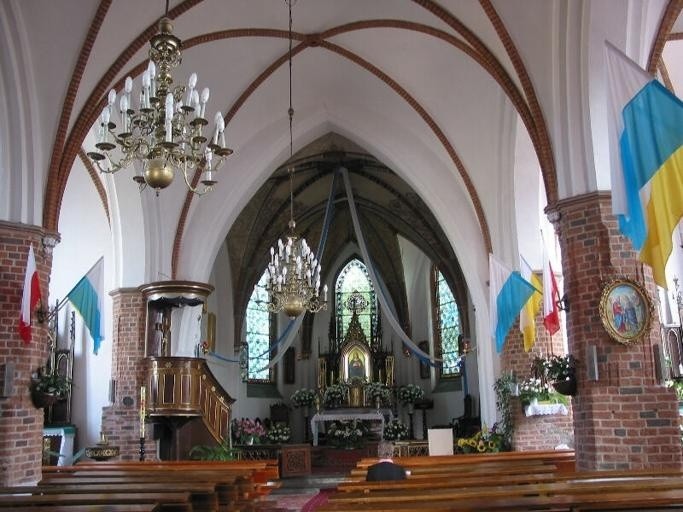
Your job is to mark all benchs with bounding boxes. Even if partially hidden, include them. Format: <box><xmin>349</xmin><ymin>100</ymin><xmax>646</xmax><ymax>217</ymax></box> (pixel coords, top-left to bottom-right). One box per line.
<box><xmin>0</xmin><ymin>460</ymin><xmax>282</xmax><ymax>511</ymax></box>
<box><xmin>312</xmin><ymin>449</ymin><xmax>683</xmax><ymax>512</ymax></box>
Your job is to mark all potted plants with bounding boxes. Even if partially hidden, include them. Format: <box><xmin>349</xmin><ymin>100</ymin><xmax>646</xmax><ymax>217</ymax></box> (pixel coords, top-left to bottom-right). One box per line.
<box><xmin>530</xmin><ymin>353</ymin><xmax>580</xmax><ymax>395</ymax></box>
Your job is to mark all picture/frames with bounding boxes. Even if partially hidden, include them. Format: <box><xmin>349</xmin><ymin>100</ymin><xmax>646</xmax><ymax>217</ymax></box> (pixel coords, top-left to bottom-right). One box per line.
<box><xmin>598</xmin><ymin>277</ymin><xmax>655</xmax><ymax>346</ymax></box>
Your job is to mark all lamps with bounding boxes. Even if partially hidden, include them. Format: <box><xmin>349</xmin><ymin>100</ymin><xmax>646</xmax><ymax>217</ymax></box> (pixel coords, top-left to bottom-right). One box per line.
<box><xmin>87</xmin><ymin>0</ymin><xmax>234</xmax><ymax>199</ymax></box>
<box><xmin>253</xmin><ymin>1</ymin><xmax>329</xmax><ymax>321</ymax></box>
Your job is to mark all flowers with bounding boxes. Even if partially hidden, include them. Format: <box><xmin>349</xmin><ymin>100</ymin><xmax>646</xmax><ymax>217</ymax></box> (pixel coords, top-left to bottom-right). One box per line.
<box><xmin>520</xmin><ymin>377</ymin><xmax>555</xmax><ymax>393</ymax></box>
<box><xmin>289</xmin><ymin>381</ymin><xmax>425</xmax><ymax>448</ymax></box>
<box><xmin>231</xmin><ymin>417</ymin><xmax>291</xmax><ymax>444</ymax></box>
<box><xmin>458</xmin><ymin>420</ymin><xmax>501</xmax><ymax>454</ymax></box>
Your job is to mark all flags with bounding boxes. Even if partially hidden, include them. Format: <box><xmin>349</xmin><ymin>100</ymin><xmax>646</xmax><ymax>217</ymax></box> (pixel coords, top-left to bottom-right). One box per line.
<box><xmin>542</xmin><ymin>241</ymin><xmax>560</xmax><ymax>336</ymax></box>
<box><xmin>67</xmin><ymin>256</ymin><xmax>105</xmax><ymax>356</ymax></box>
<box><xmin>605</xmin><ymin>44</ymin><xmax>683</xmax><ymax>290</ymax></box>
<box><xmin>489</xmin><ymin>254</ymin><xmax>537</xmax><ymax>357</ymax></box>
<box><xmin>19</xmin><ymin>242</ymin><xmax>41</xmax><ymax>344</ymax></box>
<box><xmin>519</xmin><ymin>255</ymin><xmax>542</xmax><ymax>352</ymax></box>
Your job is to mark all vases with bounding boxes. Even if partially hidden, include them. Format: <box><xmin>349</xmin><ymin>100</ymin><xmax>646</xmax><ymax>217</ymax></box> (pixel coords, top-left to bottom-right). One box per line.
<box><xmin>529</xmin><ymin>397</ymin><xmax>537</xmax><ymax>406</ymax></box>
<box><xmin>246</xmin><ymin>437</ymin><xmax>254</xmax><ymax>446</ymax></box>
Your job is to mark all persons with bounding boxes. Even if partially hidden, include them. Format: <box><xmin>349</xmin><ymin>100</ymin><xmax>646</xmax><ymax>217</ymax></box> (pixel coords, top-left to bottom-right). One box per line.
<box><xmin>366</xmin><ymin>440</ymin><xmax>412</xmax><ymax>481</ymax></box>
<box><xmin>348</xmin><ymin>351</ymin><xmax>364</xmax><ymax>377</ymax></box>
